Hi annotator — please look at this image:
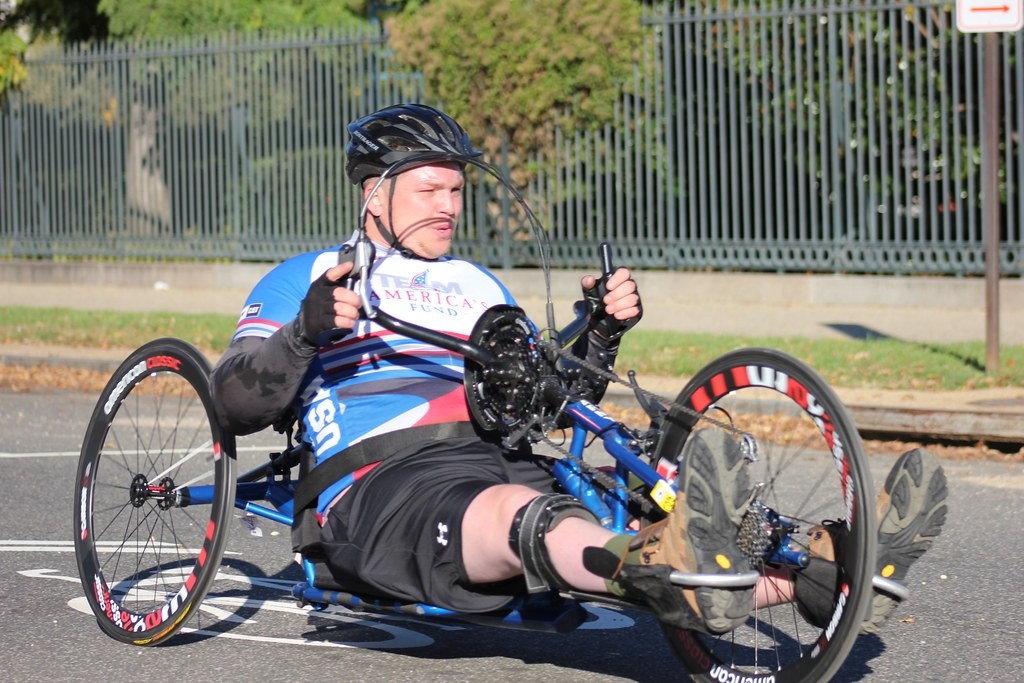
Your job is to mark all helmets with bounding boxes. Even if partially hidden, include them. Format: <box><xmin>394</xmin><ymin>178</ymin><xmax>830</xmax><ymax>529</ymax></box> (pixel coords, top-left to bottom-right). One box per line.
<box><xmin>345</xmin><ymin>104</ymin><xmax>483</xmax><ymax>184</ymax></box>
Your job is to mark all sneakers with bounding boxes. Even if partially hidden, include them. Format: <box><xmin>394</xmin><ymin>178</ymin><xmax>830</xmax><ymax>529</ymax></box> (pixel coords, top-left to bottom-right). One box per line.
<box><xmin>806</xmin><ymin>448</ymin><xmax>947</xmax><ymax>635</ymax></box>
<box><xmin>627</xmin><ymin>427</ymin><xmax>758</xmax><ymax>632</ymax></box>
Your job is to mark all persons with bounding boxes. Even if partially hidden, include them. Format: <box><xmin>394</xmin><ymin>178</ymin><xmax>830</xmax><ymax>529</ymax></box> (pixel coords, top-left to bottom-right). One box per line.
<box><xmin>208</xmin><ymin>101</ymin><xmax>949</xmax><ymax>633</ymax></box>
